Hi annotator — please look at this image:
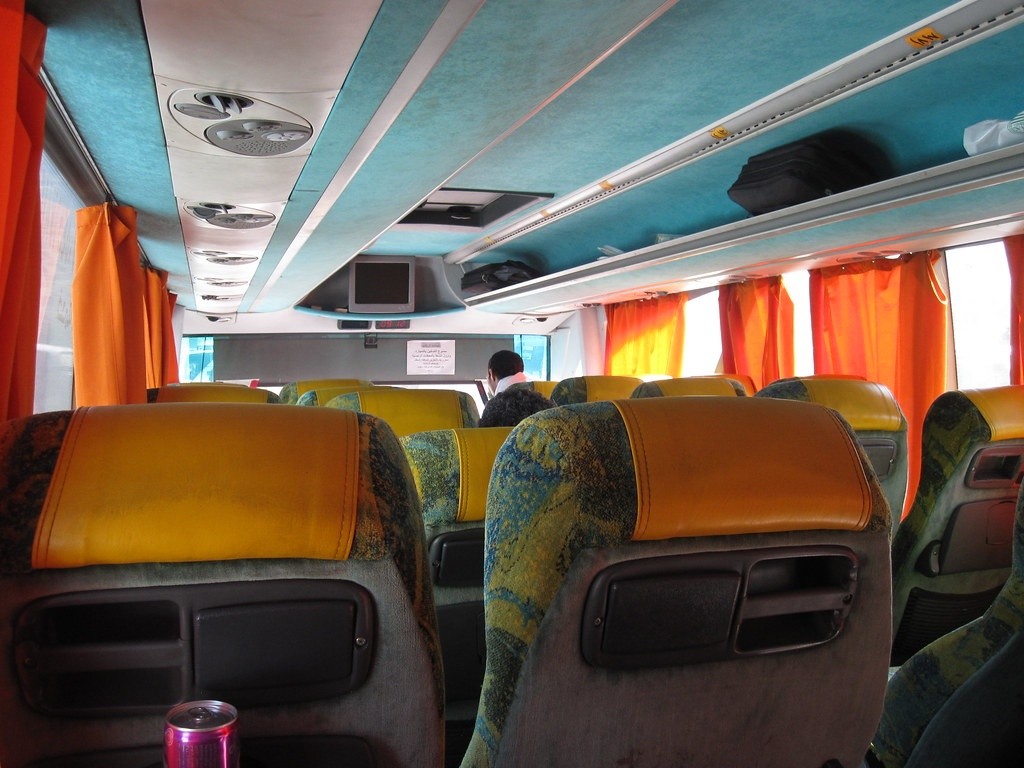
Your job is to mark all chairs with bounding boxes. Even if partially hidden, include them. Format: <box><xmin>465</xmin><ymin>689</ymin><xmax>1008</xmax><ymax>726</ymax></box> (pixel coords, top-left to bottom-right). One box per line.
<box><xmin>0</xmin><ymin>395</ymin><xmax>456</xmax><ymax>768</ymax></box>
<box><xmin>326</xmin><ymin>384</ymin><xmax>485</xmax><ymax>430</ymax></box>
<box><xmin>754</xmin><ymin>369</ymin><xmax>911</xmax><ymax>524</ymax></box>
<box><xmin>281</xmin><ymin>377</ymin><xmax>403</xmax><ymax>408</ymax></box>
<box><xmin>396</xmin><ymin>424</ymin><xmax>536</xmax><ymax>758</ymax></box>
<box><xmin>634</xmin><ymin>375</ymin><xmax>748</xmax><ymax>400</ymax></box>
<box><xmin>453</xmin><ymin>385</ymin><xmax>899</xmax><ymax>768</ymax></box>
<box><xmin>511</xmin><ymin>377</ymin><xmax>644</xmax><ymax>402</ymax></box>
<box><xmin>888</xmin><ymin>385</ymin><xmax>1024</xmax><ymax>666</ymax></box>
<box><xmin>144</xmin><ymin>376</ymin><xmax>280</xmax><ymax>406</ymax></box>
<box><xmin>873</xmin><ymin>481</ymin><xmax>1024</xmax><ymax>768</ymax></box>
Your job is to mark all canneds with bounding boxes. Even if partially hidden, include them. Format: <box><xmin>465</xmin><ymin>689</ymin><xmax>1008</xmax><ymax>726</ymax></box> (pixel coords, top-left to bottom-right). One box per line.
<box><xmin>162</xmin><ymin>701</ymin><xmax>242</xmax><ymax>768</ymax></box>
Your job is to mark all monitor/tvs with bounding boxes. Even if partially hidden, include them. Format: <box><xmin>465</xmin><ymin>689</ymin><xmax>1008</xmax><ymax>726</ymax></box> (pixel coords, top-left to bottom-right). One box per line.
<box><xmin>348</xmin><ymin>255</ymin><xmax>415</xmax><ymax>313</ymax></box>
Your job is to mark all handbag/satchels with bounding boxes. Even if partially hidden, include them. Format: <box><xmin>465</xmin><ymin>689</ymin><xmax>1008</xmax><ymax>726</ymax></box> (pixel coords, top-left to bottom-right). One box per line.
<box><xmin>461</xmin><ymin>258</ymin><xmax>543</xmax><ymax>296</ymax></box>
<box><xmin>724</xmin><ymin>127</ymin><xmax>893</xmax><ymax>218</ymax></box>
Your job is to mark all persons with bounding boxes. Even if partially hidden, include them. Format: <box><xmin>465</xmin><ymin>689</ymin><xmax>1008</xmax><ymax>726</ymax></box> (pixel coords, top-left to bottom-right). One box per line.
<box><xmin>486</xmin><ymin>350</ymin><xmax>526</xmax><ymax>396</ymax></box>
